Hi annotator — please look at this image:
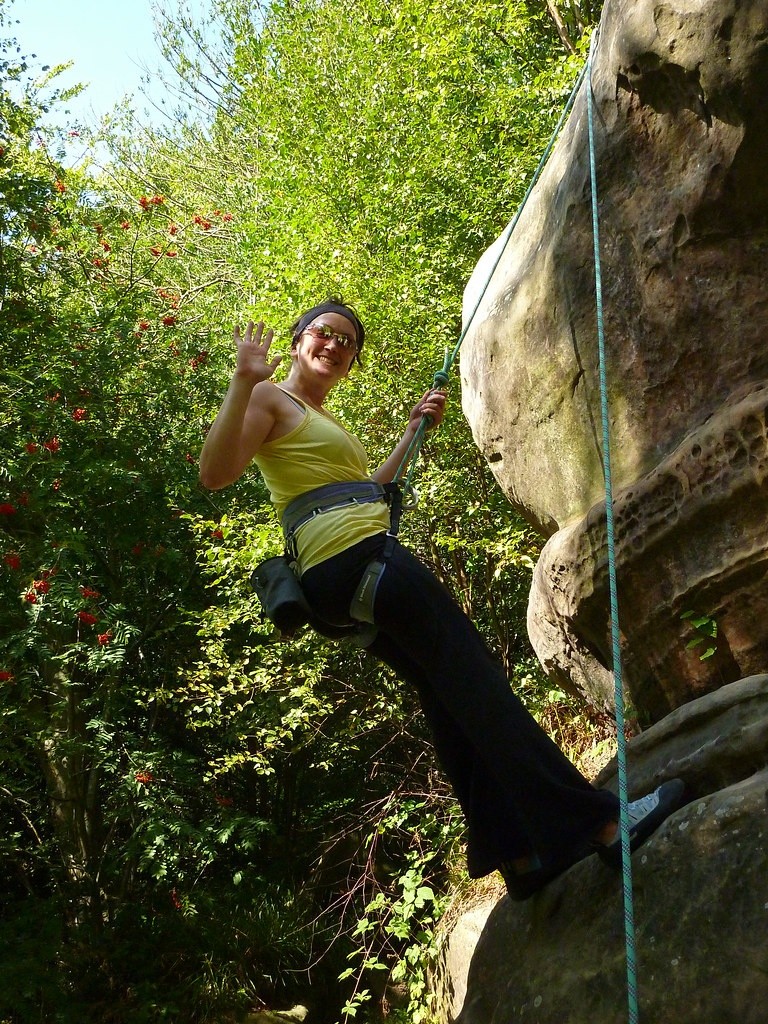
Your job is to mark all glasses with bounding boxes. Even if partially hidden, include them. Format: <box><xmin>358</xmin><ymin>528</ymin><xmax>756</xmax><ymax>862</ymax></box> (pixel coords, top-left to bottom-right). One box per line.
<box><xmin>304</xmin><ymin>322</ymin><xmax>359</xmax><ymax>356</ymax></box>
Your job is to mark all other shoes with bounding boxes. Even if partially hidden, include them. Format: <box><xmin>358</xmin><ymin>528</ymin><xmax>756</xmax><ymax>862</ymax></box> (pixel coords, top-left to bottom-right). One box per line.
<box><xmin>498</xmin><ymin>837</ymin><xmax>601</xmax><ymax>901</ymax></box>
<box><xmin>598</xmin><ymin>778</ymin><xmax>685</xmax><ymax>872</ymax></box>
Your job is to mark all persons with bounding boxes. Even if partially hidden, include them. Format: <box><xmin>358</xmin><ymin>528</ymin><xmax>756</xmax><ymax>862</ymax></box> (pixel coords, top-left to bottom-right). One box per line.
<box><xmin>200</xmin><ymin>292</ymin><xmax>685</xmax><ymax>902</ymax></box>
